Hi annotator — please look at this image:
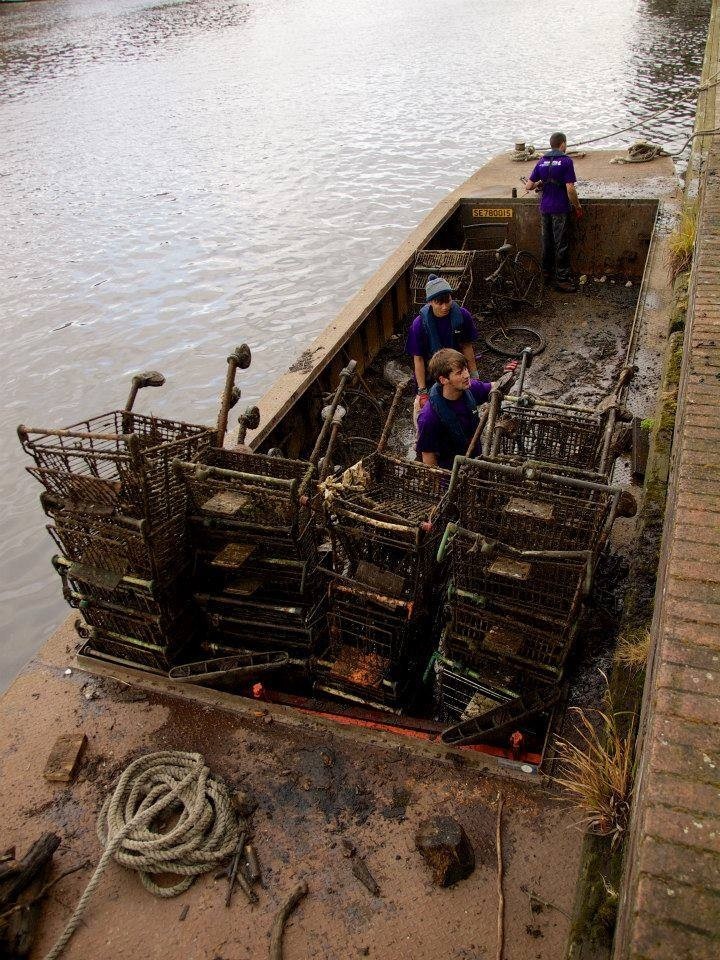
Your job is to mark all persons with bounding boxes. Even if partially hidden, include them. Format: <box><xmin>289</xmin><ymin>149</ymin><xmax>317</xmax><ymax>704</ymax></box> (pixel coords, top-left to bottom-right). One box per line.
<box><xmin>407</xmin><ymin>274</ymin><xmax>481</xmax><ymax>410</ymax></box>
<box><xmin>525</xmin><ymin>131</ymin><xmax>584</xmax><ymax>292</ymax></box>
<box><xmin>415</xmin><ymin>349</ymin><xmax>519</xmax><ymax>472</ymax></box>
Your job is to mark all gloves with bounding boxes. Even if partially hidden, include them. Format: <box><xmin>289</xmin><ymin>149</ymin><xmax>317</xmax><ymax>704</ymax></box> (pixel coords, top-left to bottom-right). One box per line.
<box><xmin>469</xmin><ymin>371</ymin><xmax>479</xmax><ymax>382</ymax></box>
<box><xmin>418</xmin><ymin>387</ymin><xmax>429</xmax><ymax>409</ymax></box>
<box><xmin>576</xmin><ymin>207</ymin><xmax>584</xmax><ymax>219</ymax></box>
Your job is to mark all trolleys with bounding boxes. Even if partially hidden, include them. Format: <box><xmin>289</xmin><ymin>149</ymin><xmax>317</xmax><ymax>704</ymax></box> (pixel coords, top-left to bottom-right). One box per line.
<box><xmin>18</xmin><ymin>345</ymin><xmax>621</xmax><ymax>735</ymax></box>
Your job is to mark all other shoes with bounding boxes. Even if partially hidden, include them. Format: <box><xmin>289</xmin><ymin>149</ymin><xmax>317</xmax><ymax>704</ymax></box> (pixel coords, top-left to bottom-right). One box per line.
<box><xmin>555</xmin><ymin>281</ymin><xmax>575</xmax><ymax>293</ymax></box>
<box><xmin>543</xmin><ymin>270</ymin><xmax>551</xmax><ymax>279</ymax></box>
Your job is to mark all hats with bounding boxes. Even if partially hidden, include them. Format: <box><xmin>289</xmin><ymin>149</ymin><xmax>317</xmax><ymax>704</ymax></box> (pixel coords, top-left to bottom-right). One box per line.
<box><xmin>426</xmin><ymin>274</ymin><xmax>452</xmax><ymax>301</ymax></box>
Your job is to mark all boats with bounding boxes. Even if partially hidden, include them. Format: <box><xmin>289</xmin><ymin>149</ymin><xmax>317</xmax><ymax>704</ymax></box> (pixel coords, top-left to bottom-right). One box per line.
<box><xmin>1</xmin><ymin>145</ymin><xmax>680</xmax><ymax>960</ymax></box>
